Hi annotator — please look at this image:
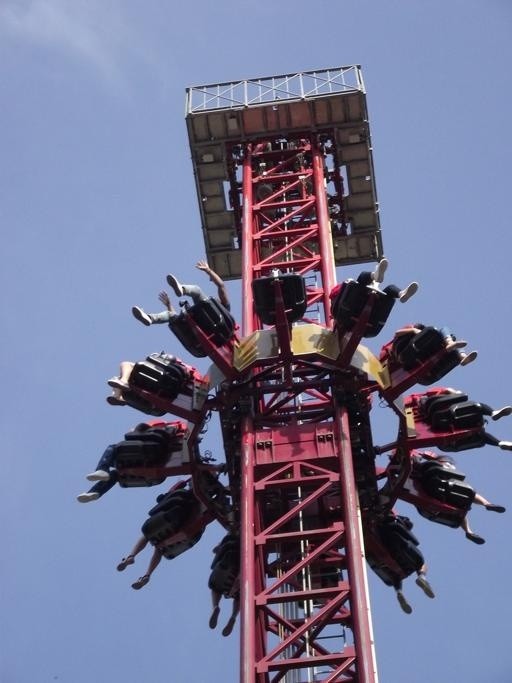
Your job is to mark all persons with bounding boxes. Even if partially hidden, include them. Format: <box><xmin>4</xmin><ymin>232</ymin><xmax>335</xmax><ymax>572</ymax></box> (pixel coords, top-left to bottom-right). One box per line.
<box><xmin>428</xmin><ymin>394</ymin><xmax>512</xmax><ymax>451</ymax></box>
<box><xmin>106</xmin><ymin>353</ymin><xmax>182</xmax><ymax>406</ymax></box>
<box><xmin>435</xmin><ymin>456</ymin><xmax>505</xmax><ymax>544</ymax></box>
<box><xmin>384</xmin><ymin>516</ymin><xmax>434</xmax><ymax>613</ymax></box>
<box><xmin>330</xmin><ymin>259</ymin><xmax>418</xmax><ymax>303</ymax></box>
<box><xmin>396</xmin><ymin>324</ymin><xmax>478</xmax><ymax>365</ymax></box>
<box><xmin>77</xmin><ymin>423</ymin><xmax>173</xmax><ymax>503</ymax></box>
<box><xmin>115</xmin><ymin>492</ymin><xmax>189</xmax><ymax>589</ymax></box>
<box><xmin>209</xmin><ymin>534</ymin><xmax>240</xmax><ymax>637</ymax></box>
<box><xmin>133</xmin><ymin>260</ymin><xmax>229</xmax><ymax>325</ymax></box>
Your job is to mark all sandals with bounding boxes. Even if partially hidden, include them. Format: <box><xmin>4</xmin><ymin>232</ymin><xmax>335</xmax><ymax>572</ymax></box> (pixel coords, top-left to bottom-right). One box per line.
<box><xmin>117</xmin><ymin>555</ymin><xmax>150</xmax><ymax>590</ymax></box>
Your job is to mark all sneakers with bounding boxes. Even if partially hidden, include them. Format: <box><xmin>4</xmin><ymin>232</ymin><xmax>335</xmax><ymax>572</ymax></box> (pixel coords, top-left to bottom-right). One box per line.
<box><xmin>106</xmin><ymin>379</ymin><xmax>130</xmax><ymax>405</ymax></box>
<box><xmin>374</xmin><ymin>259</ymin><xmax>389</xmax><ymax>283</ymax></box>
<box><xmin>416</xmin><ymin>577</ymin><xmax>435</xmax><ymax>599</ymax></box>
<box><xmin>131</xmin><ymin>306</ymin><xmax>152</xmax><ymax>326</ymax></box>
<box><xmin>486</xmin><ymin>504</ymin><xmax>505</xmax><ymax>513</ymax></box>
<box><xmin>399</xmin><ymin>282</ymin><xmax>419</xmax><ymax>303</ymax></box>
<box><xmin>166</xmin><ymin>274</ymin><xmax>183</xmax><ymax>297</ymax></box>
<box><xmin>466</xmin><ymin>533</ymin><xmax>485</xmax><ymax>544</ymax></box>
<box><xmin>499</xmin><ymin>441</ymin><xmax>512</xmax><ymax>451</ymax></box>
<box><xmin>491</xmin><ymin>406</ymin><xmax>512</xmax><ymax>421</ymax></box>
<box><xmin>397</xmin><ymin>592</ymin><xmax>413</xmax><ymax>614</ymax></box>
<box><xmin>446</xmin><ymin>341</ymin><xmax>477</xmax><ymax>366</ymax></box>
<box><xmin>208</xmin><ymin>606</ymin><xmax>236</xmax><ymax>637</ymax></box>
<box><xmin>76</xmin><ymin>470</ymin><xmax>110</xmax><ymax>503</ymax></box>
